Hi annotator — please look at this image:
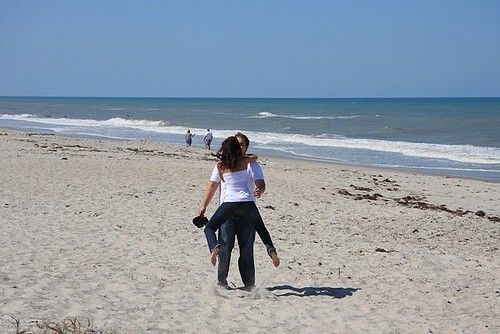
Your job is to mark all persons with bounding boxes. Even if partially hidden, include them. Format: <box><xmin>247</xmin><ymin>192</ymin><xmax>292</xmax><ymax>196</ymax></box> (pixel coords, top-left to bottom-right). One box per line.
<box><xmin>198</xmin><ymin>132</ymin><xmax>265</xmax><ymax>293</ymax></box>
<box><xmin>203</xmin><ymin>136</ymin><xmax>279</xmax><ymax>267</ymax></box>
<box><xmin>185</xmin><ymin>130</ymin><xmax>195</xmax><ymax>146</ymax></box>
<box><xmin>202</xmin><ymin>128</ymin><xmax>213</xmax><ymax>150</ymax></box>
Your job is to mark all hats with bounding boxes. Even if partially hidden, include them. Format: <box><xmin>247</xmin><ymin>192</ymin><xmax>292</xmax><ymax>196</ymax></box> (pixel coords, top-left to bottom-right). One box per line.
<box><xmin>193</xmin><ymin>216</ymin><xmax>208</xmax><ymax>228</ymax></box>
